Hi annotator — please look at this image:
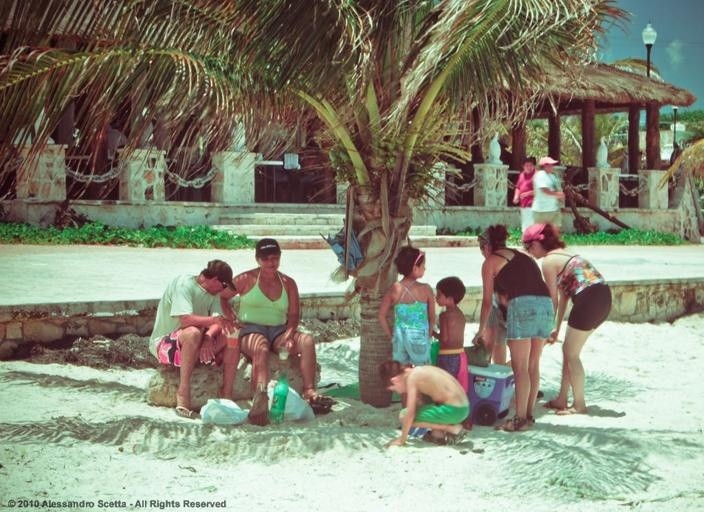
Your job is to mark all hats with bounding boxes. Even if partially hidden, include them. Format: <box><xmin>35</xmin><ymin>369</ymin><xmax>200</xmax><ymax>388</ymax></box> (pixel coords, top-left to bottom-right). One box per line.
<box><xmin>539</xmin><ymin>156</ymin><xmax>559</xmax><ymax>165</ymax></box>
<box><xmin>207</xmin><ymin>260</ymin><xmax>237</xmax><ymax>292</ymax></box>
<box><xmin>256</xmin><ymin>238</ymin><xmax>281</xmax><ymax>256</ymax></box>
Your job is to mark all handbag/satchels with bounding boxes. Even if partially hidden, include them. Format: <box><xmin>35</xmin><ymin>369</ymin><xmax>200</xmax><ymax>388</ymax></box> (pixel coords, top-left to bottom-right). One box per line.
<box><xmin>327</xmin><ymin>226</ymin><xmax>362</xmax><ymax>272</ymax></box>
<box><xmin>464</xmin><ymin>340</ymin><xmax>489</xmax><ymax>367</ymax></box>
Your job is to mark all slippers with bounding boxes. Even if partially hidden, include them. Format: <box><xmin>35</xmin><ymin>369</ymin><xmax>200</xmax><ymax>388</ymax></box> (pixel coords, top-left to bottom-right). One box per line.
<box><xmin>303</xmin><ymin>394</ymin><xmax>337</xmax><ymax>405</ymax></box>
<box><xmin>556</xmin><ymin>407</ymin><xmax>589</xmax><ymax>415</ymax></box>
<box><xmin>543</xmin><ymin>401</ymin><xmax>567</xmax><ymax>409</ymax></box>
<box><xmin>176</xmin><ymin>406</ymin><xmax>196</xmax><ymax>418</ymax></box>
<box><xmin>443</xmin><ymin>430</ymin><xmax>467</xmax><ymax>444</ymax></box>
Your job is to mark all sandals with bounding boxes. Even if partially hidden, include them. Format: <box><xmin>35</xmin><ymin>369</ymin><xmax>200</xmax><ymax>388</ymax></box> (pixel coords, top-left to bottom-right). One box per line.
<box><xmin>527</xmin><ymin>416</ymin><xmax>536</xmax><ymax>426</ymax></box>
<box><xmin>496</xmin><ymin>415</ymin><xmax>528</xmax><ymax>431</ymax></box>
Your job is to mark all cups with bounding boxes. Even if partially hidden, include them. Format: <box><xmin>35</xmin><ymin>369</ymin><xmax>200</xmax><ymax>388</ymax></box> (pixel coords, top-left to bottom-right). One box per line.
<box><xmin>278</xmin><ymin>346</ymin><xmax>289</xmax><ymax>360</ymax></box>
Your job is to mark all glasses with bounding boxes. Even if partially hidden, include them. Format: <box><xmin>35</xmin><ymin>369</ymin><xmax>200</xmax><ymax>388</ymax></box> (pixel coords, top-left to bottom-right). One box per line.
<box><xmin>217</xmin><ymin>279</ymin><xmax>228</xmax><ymax>289</ymax></box>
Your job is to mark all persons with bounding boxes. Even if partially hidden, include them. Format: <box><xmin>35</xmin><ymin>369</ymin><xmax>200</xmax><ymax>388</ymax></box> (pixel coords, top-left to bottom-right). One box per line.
<box><xmin>532</xmin><ymin>156</ymin><xmax>564</xmax><ymax>234</ymax></box>
<box><xmin>485</xmin><ymin>295</ymin><xmax>544</xmax><ymax>396</ymax></box>
<box><xmin>378</xmin><ymin>244</ymin><xmax>436</xmax><ymax>407</ymax></box>
<box><xmin>522</xmin><ymin>222</ymin><xmax>611</xmax><ymax>416</ymax></box>
<box><xmin>220</xmin><ymin>239</ymin><xmax>335</xmax><ymax>426</ymax></box>
<box><xmin>379</xmin><ymin>361</ymin><xmax>470</xmax><ymax>448</ymax></box>
<box><xmin>146</xmin><ymin>260</ymin><xmax>239</xmax><ymax>419</ymax></box>
<box><xmin>433</xmin><ymin>276</ymin><xmax>472</xmax><ymax>430</ymax></box>
<box><xmin>472</xmin><ymin>224</ymin><xmax>554</xmax><ymax>430</ymax></box>
<box><xmin>513</xmin><ymin>156</ymin><xmax>537</xmax><ymax>230</ymax></box>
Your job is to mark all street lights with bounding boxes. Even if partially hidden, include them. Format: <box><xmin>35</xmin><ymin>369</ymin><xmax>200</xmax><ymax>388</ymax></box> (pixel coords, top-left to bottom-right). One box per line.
<box><xmin>672</xmin><ymin>102</ymin><xmax>679</xmax><ymax>146</ymax></box>
<box><xmin>642</xmin><ymin>22</ymin><xmax>657</xmax><ymax>77</ymax></box>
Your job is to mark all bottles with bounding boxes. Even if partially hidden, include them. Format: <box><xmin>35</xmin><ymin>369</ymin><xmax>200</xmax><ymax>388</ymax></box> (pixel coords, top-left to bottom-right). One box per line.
<box><xmin>269</xmin><ymin>372</ymin><xmax>289</xmax><ymax>423</ymax></box>
